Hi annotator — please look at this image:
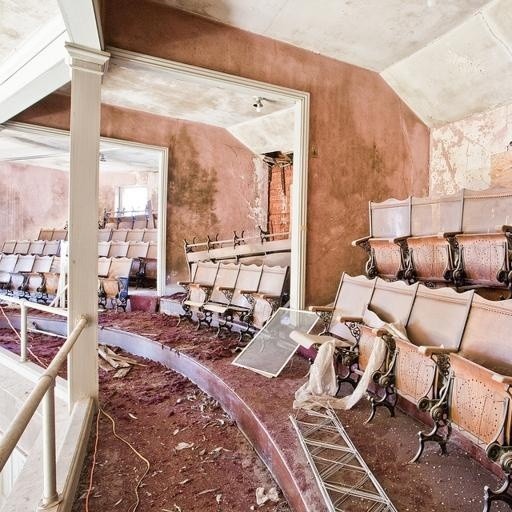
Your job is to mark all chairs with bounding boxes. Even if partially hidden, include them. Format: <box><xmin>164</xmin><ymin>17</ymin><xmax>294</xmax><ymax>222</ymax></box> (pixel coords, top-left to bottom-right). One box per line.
<box><xmin>410</xmin><ymin>292</ymin><xmax>512</xmax><ymax>512</ymax></box>
<box><xmin>351</xmin><ymin>188</ymin><xmax>512</xmax><ymax>301</ymax></box>
<box><xmin>289</xmin><ymin>269</ymin><xmax>474</xmax><ymax>461</ymax></box>
<box><xmin>174</xmin><ymin>260</ymin><xmax>290</xmax><ymax>343</ymax></box>
<box><xmin>0</xmin><ymin>215</ymin><xmax>158</xmax><ymax>311</ymax></box>
